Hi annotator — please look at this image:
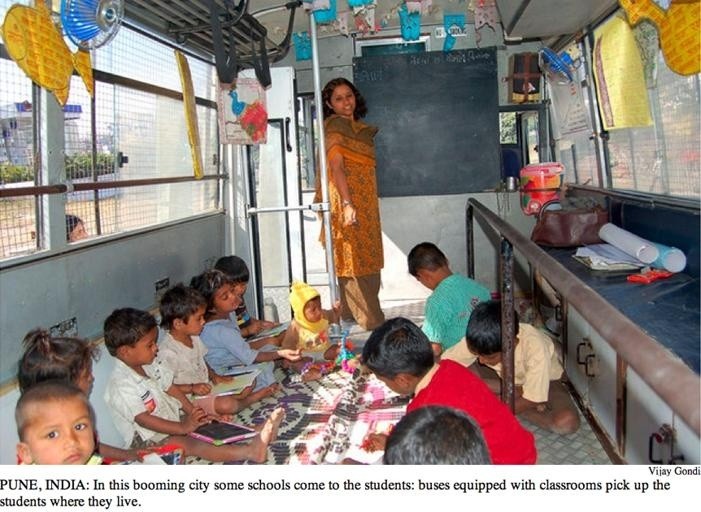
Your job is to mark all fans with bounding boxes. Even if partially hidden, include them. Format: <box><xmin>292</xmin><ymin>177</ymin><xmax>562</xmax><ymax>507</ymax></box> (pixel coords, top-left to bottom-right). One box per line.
<box><xmin>59</xmin><ymin>1</ymin><xmax>125</xmax><ymax>52</ymax></box>
<box><xmin>535</xmin><ymin>42</ymin><xmax>582</xmax><ymax>86</ymax></box>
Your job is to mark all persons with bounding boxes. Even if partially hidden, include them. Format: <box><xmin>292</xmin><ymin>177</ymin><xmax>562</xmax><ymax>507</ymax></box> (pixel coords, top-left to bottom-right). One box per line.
<box><xmin>647</xmin><ymin>157</ymin><xmax>663</xmax><ymax>191</ymax></box>
<box><xmin>311</xmin><ymin>77</ymin><xmax>387</xmax><ymax>331</ymax></box>
<box><xmin>1</xmin><ymin>212</ymin><xmax>580</xmax><ymax>465</ymax></box>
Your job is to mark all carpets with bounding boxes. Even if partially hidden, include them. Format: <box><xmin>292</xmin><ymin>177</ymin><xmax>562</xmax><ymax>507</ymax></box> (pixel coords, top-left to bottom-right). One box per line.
<box><xmin>181</xmin><ymin>354</ymin><xmax>410</xmax><ymax>465</ymax></box>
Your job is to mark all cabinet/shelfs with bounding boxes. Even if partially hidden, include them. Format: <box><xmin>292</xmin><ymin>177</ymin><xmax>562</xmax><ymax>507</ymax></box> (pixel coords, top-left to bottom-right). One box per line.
<box><xmin>623</xmin><ymin>363</ymin><xmax>701</xmax><ymax>464</ymax></box>
<box><xmin>535</xmin><ymin>268</ymin><xmax>566</xmax><ymax>364</ymax></box>
<box><xmin>567</xmin><ymin>300</ymin><xmax>622</xmax><ymax>447</ymax></box>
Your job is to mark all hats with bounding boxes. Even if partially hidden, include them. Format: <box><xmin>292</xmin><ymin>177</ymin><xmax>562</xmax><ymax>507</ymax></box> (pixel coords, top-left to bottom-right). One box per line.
<box><xmin>288</xmin><ymin>282</ymin><xmax>328</xmax><ymax>334</ymax></box>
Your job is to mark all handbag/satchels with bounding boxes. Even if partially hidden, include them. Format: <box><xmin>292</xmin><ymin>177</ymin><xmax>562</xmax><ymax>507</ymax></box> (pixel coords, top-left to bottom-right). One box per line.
<box><xmin>531</xmin><ymin>199</ymin><xmax>608</xmax><ymax>248</ymax></box>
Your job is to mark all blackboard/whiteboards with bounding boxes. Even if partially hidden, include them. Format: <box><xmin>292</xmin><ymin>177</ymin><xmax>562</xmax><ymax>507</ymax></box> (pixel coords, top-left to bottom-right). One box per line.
<box><xmin>352</xmin><ymin>46</ymin><xmax>501</xmax><ymax>197</ymax></box>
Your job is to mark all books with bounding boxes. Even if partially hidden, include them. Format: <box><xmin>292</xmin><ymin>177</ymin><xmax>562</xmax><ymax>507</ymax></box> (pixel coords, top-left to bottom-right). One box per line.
<box><xmin>572</xmin><ymin>241</ymin><xmax>643</xmax><ymax>271</ymax></box>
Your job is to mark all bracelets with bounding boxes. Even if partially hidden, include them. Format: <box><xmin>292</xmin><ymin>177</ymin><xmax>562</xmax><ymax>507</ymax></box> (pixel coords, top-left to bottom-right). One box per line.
<box><xmin>341</xmin><ymin>199</ymin><xmax>353</xmax><ymax>207</ymax></box>
